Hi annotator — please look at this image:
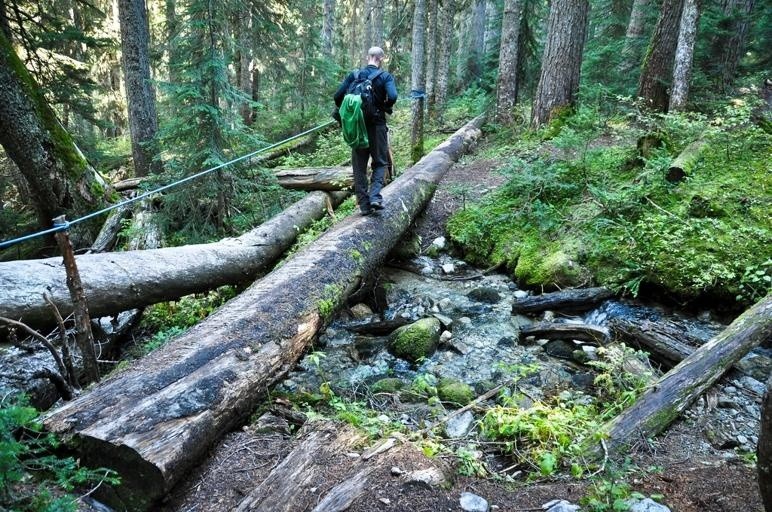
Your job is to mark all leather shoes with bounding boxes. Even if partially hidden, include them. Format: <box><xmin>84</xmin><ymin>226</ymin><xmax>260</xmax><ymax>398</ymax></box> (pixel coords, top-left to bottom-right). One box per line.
<box><xmin>359</xmin><ymin>202</ymin><xmax>385</xmax><ymax>216</ymax></box>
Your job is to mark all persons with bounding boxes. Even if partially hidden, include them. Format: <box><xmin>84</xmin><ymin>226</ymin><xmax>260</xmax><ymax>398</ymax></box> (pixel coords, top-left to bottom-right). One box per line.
<box><xmin>334</xmin><ymin>46</ymin><xmax>398</xmax><ymax>216</ymax></box>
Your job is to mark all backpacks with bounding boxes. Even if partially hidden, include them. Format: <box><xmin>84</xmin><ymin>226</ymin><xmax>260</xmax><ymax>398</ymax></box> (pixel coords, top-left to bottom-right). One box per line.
<box><xmin>344</xmin><ymin>69</ymin><xmax>386</xmax><ymax>126</ymax></box>
